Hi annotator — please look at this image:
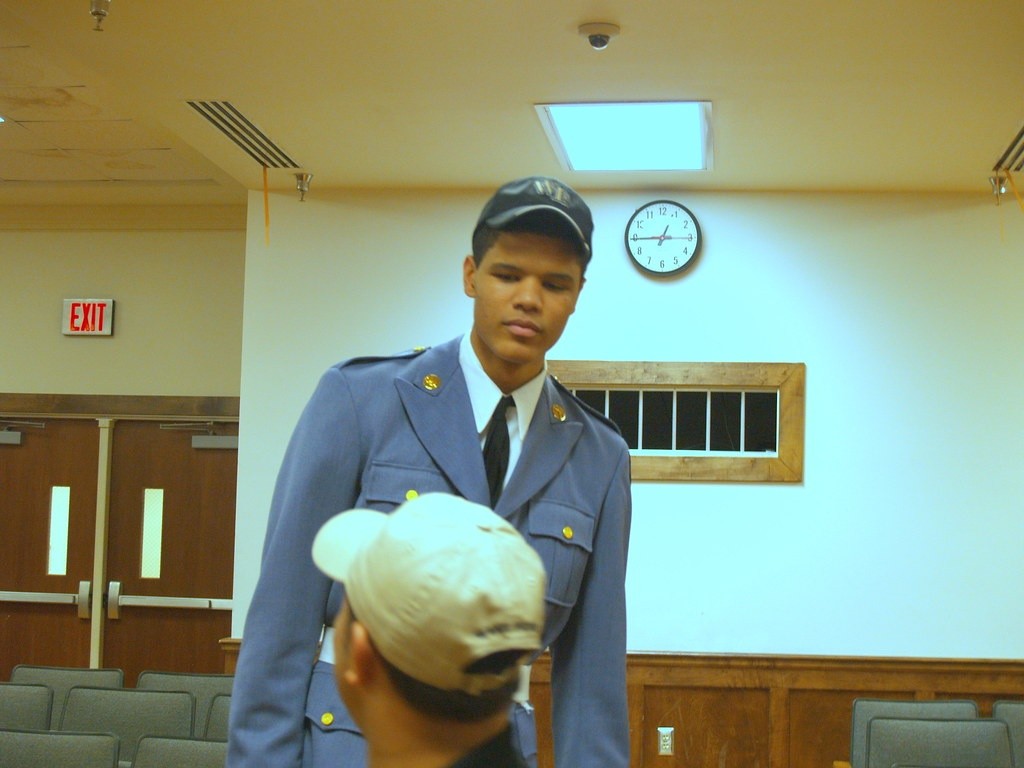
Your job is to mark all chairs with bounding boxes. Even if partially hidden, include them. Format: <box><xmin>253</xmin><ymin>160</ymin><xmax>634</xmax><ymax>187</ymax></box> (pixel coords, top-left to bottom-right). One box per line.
<box><xmin>849</xmin><ymin>696</ymin><xmax>1024</xmax><ymax>768</ymax></box>
<box><xmin>0</xmin><ymin>662</ymin><xmax>235</xmax><ymax>768</ymax></box>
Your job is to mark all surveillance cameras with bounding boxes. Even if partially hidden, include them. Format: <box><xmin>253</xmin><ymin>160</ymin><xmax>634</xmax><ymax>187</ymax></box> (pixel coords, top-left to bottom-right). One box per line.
<box><xmin>588</xmin><ymin>34</ymin><xmax>611</xmax><ymax>50</ymax></box>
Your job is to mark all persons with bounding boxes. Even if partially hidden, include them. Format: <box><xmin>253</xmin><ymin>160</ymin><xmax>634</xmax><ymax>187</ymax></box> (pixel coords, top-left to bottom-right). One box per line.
<box><xmin>331</xmin><ymin>499</ymin><xmax>549</xmax><ymax>768</ymax></box>
<box><xmin>222</xmin><ymin>175</ymin><xmax>632</xmax><ymax>768</ymax></box>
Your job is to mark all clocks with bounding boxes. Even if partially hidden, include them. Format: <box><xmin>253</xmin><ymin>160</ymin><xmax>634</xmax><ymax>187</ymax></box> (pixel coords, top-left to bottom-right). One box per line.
<box><xmin>624</xmin><ymin>199</ymin><xmax>703</xmax><ymax>281</ymax></box>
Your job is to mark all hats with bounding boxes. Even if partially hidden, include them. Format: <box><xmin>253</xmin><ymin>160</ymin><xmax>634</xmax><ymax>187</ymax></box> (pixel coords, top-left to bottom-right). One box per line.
<box><xmin>477</xmin><ymin>176</ymin><xmax>594</xmax><ymax>267</ymax></box>
<box><xmin>311</xmin><ymin>492</ymin><xmax>548</xmax><ymax>697</ymax></box>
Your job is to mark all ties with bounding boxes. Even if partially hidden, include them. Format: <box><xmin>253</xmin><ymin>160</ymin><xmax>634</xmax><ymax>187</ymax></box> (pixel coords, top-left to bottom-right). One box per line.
<box><xmin>483</xmin><ymin>394</ymin><xmax>515</xmax><ymax>510</ymax></box>
